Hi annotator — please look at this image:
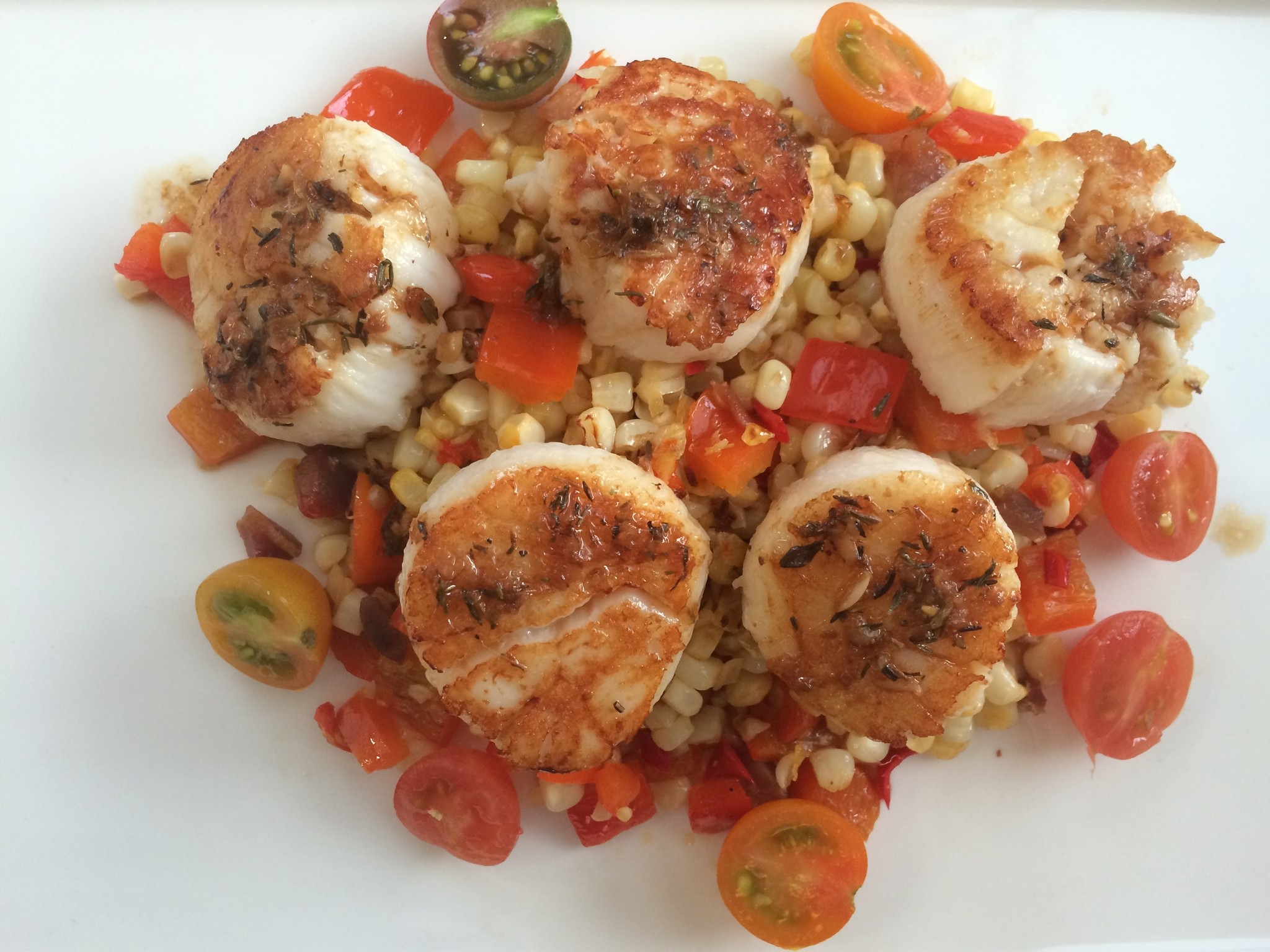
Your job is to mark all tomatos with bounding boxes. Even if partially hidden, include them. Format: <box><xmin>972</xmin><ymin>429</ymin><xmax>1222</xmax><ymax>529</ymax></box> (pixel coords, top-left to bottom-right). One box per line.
<box><xmin>116</xmin><ymin>0</ymin><xmax>1217</xmax><ymax>948</ymax></box>
